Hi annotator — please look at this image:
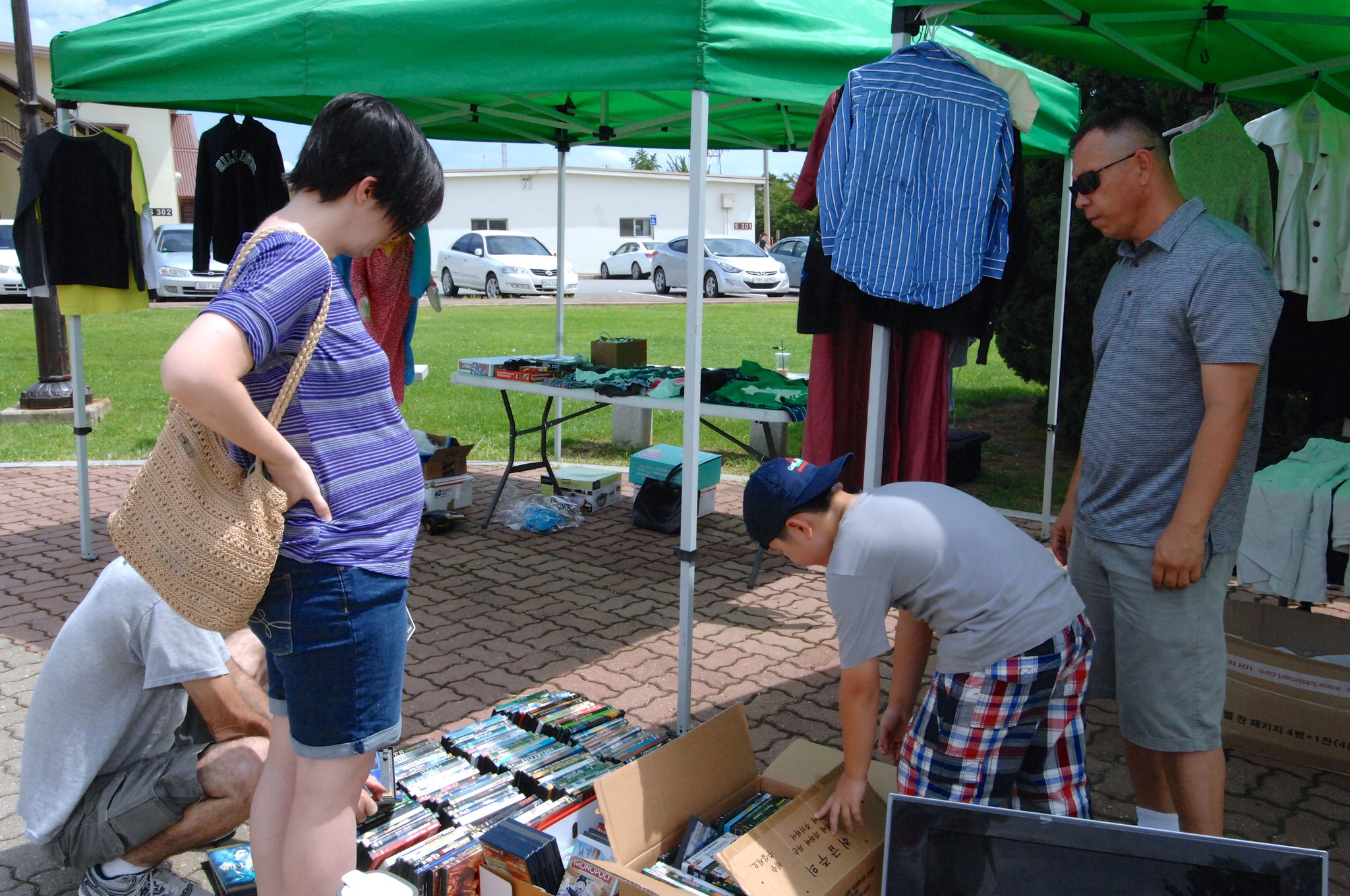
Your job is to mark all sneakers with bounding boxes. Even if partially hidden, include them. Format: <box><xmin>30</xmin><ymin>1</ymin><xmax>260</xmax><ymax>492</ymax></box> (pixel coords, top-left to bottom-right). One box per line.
<box><xmin>79</xmin><ymin>857</ymin><xmax>216</xmax><ymax>896</ymax></box>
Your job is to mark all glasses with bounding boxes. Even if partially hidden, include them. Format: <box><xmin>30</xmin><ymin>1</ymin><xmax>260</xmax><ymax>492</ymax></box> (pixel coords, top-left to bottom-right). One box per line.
<box><xmin>1068</xmin><ymin>145</ymin><xmax>1156</xmax><ymax>200</ymax></box>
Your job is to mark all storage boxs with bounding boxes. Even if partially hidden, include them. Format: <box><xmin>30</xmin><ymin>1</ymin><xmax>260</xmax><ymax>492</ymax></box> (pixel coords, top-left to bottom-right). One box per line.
<box><xmin>1215</xmin><ymin>599</ymin><xmax>1350</xmax><ymax>776</ymax></box>
<box><xmin>457</xmin><ymin>354</ymin><xmax>581</xmax><ymax>382</ymax></box>
<box><xmin>540</xmin><ymin>466</ymin><xmax>622</xmax><ymax>512</ymax></box>
<box><xmin>591</xmin><ymin>337</ymin><xmax>647</xmax><ymax>369</ymax></box>
<box><xmin>947</xmin><ymin>425</ymin><xmax>993</xmax><ymax>483</ymax></box>
<box><xmin>574</xmin><ymin>701</ymin><xmax>896</xmax><ymax>896</ymax></box>
<box><xmin>417</xmin><ymin>433</ymin><xmax>474</xmax><ymax>511</ymax></box>
<box><xmin>629</xmin><ymin>443</ymin><xmax>722</xmax><ymax>518</ymax></box>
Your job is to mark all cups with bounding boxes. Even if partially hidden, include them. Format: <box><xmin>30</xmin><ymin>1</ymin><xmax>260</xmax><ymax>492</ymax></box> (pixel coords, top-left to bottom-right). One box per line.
<box><xmin>775</xmin><ymin>352</ymin><xmax>791</xmax><ymax>376</ymax></box>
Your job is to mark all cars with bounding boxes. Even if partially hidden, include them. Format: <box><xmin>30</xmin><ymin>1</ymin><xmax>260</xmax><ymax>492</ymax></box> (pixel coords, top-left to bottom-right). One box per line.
<box><xmin>435</xmin><ymin>229</ymin><xmax>580</xmax><ymax>300</ymax></box>
<box><xmin>766</xmin><ymin>235</ymin><xmax>811</xmax><ymax>289</ymax></box>
<box><xmin>651</xmin><ymin>235</ymin><xmax>790</xmax><ymax>297</ymax></box>
<box><xmin>148</xmin><ymin>223</ymin><xmax>231</xmax><ymax>303</ymax></box>
<box><xmin>600</xmin><ymin>238</ymin><xmax>668</xmax><ymax>280</ymax></box>
<box><xmin>0</xmin><ymin>218</ymin><xmax>30</xmax><ymax>297</ymax></box>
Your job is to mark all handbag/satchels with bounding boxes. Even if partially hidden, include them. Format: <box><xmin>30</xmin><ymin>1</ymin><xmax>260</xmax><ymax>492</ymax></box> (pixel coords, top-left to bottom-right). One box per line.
<box><xmin>107</xmin><ymin>225</ymin><xmax>330</xmax><ymax>632</ymax></box>
<box><xmin>632</xmin><ymin>463</ymin><xmax>701</xmax><ymax>537</ymax></box>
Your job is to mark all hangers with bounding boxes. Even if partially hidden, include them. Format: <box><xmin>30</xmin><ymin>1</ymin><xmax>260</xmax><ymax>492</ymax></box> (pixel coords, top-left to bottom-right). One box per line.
<box><xmin>22</xmin><ymin>100</ymin><xmax>136</xmax><ymax>155</ymax></box>
<box><xmin>200</xmin><ymin>112</ymin><xmax>277</xmax><ymax>143</ymax></box>
<box><xmin>826</xmin><ymin>3</ymin><xmax>1031</xmax><ymax>114</ymax></box>
<box><xmin>1162</xmin><ymin>70</ymin><xmax>1350</xmax><ymax>164</ymax></box>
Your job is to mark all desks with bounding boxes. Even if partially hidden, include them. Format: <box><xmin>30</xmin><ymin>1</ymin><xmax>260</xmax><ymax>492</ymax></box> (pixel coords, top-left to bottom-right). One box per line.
<box><xmin>452</xmin><ymin>368</ymin><xmax>809</xmax><ymax>588</ymax></box>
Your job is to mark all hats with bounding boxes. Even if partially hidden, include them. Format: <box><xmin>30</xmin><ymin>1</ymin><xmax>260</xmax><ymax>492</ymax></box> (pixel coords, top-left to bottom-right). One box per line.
<box><xmin>742</xmin><ymin>453</ymin><xmax>858</xmax><ymax>551</ymax></box>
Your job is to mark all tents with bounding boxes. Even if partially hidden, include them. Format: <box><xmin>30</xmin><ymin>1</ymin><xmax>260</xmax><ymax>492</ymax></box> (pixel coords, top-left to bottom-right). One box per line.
<box><xmin>47</xmin><ymin>1</ymin><xmax>1082</xmax><ymax>737</ymax></box>
<box><xmin>861</xmin><ymin>0</ymin><xmax>1350</xmax><ymax>499</ymax></box>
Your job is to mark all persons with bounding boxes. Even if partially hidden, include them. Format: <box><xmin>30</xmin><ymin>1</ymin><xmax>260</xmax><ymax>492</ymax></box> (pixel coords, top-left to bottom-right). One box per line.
<box><xmin>758</xmin><ymin>233</ymin><xmax>769</xmax><ymax>251</ymax></box>
<box><xmin>160</xmin><ymin>93</ymin><xmax>444</xmax><ymax>896</ymax></box>
<box><xmin>16</xmin><ymin>552</ymin><xmax>387</xmax><ymax>896</ymax></box>
<box><xmin>743</xmin><ymin>455</ymin><xmax>1096</xmax><ymax>833</ymax></box>
<box><xmin>1052</xmin><ymin>105</ymin><xmax>1282</xmax><ymax>839</ymax></box>
